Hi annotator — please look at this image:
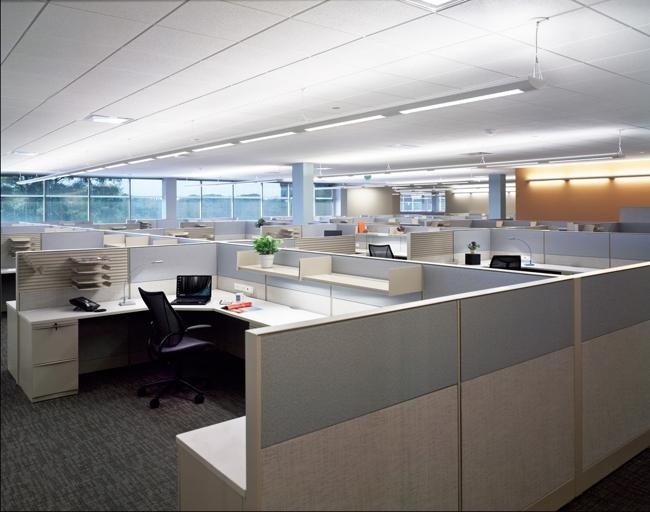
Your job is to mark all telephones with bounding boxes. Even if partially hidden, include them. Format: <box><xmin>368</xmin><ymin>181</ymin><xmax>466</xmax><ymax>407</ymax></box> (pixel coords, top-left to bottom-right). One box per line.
<box><xmin>69</xmin><ymin>297</ymin><xmax>100</xmax><ymax>312</ymax></box>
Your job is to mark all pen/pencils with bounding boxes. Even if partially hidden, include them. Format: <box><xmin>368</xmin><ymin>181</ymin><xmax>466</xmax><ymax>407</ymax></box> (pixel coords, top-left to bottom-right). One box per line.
<box><xmin>222</xmin><ymin>303</ymin><xmax>251</xmax><ymax>309</ymax></box>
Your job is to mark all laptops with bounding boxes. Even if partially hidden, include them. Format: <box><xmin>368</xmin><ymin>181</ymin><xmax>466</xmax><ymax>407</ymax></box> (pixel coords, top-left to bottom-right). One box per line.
<box><xmin>170</xmin><ymin>274</ymin><xmax>212</xmax><ymax>306</ymax></box>
